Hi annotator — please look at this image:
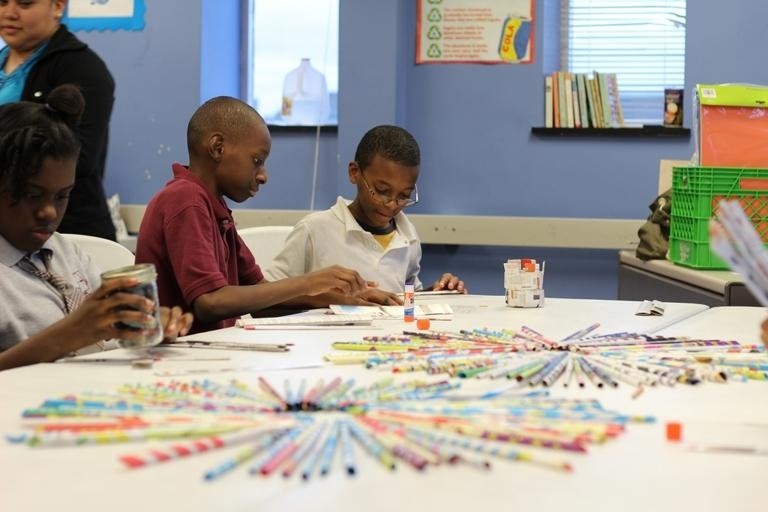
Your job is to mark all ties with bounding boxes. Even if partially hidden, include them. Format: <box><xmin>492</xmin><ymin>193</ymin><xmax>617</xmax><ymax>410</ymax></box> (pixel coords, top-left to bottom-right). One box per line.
<box><xmin>16</xmin><ymin>249</ymin><xmax>87</xmax><ymax>313</ymax></box>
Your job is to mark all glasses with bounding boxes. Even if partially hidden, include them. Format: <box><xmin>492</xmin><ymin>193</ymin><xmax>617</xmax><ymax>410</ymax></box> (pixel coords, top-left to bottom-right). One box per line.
<box><xmin>357</xmin><ymin>172</ymin><xmax>420</xmax><ymax>206</ymax></box>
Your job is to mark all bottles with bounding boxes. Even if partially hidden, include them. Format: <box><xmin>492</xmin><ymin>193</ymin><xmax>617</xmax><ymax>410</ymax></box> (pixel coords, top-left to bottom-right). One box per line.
<box><xmin>102</xmin><ymin>263</ymin><xmax>164</xmax><ymax>351</ymax></box>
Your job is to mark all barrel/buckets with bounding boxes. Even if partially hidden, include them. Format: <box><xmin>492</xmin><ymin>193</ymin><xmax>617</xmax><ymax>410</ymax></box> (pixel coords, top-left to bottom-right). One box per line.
<box><xmin>280</xmin><ymin>57</ymin><xmax>331</xmax><ymax>126</ymax></box>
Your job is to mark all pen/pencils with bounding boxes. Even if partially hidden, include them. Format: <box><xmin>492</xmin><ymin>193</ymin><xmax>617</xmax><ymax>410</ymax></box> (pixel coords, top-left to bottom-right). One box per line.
<box><xmin>3</xmin><ymin>323</ymin><xmax>768</xmax><ymax>481</ymax></box>
<box><xmin>708</xmin><ymin>195</ymin><xmax>768</xmax><ymax>307</ymax></box>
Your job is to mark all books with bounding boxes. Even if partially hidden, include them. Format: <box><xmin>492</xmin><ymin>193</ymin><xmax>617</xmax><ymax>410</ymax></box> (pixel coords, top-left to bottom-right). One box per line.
<box><xmin>545</xmin><ymin>68</ymin><xmax>627</xmax><ymax>130</ymax></box>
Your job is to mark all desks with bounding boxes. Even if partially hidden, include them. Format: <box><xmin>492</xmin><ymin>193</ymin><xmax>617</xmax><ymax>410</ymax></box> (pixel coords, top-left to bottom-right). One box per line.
<box><xmin>0</xmin><ymin>287</ymin><xmax>768</xmax><ymax>512</ymax></box>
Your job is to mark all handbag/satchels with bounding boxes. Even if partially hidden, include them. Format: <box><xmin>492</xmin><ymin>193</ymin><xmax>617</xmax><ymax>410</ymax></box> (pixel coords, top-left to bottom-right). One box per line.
<box><xmin>635</xmin><ymin>186</ymin><xmax>672</xmax><ymax>258</ymax></box>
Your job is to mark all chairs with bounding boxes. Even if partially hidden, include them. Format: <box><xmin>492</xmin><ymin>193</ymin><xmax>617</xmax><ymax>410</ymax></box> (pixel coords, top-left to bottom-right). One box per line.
<box><xmin>237</xmin><ymin>224</ymin><xmax>296</xmax><ymax>278</ymax></box>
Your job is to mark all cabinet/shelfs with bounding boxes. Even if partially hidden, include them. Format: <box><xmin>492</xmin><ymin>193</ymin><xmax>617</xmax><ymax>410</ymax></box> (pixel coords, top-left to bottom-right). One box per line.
<box><xmin>616</xmin><ymin>247</ymin><xmax>763</xmax><ymax>308</ymax></box>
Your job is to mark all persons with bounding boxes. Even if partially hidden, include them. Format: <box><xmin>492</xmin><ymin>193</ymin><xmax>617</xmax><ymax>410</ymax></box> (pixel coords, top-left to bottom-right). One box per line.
<box><xmin>0</xmin><ymin>0</ymin><xmax>121</xmax><ymax>245</ymax></box>
<box><xmin>135</xmin><ymin>95</ymin><xmax>405</xmax><ymax>341</ymax></box>
<box><xmin>0</xmin><ymin>85</ymin><xmax>196</xmax><ymax>370</ymax></box>
<box><xmin>248</xmin><ymin>125</ymin><xmax>469</xmax><ymax>318</ymax></box>
